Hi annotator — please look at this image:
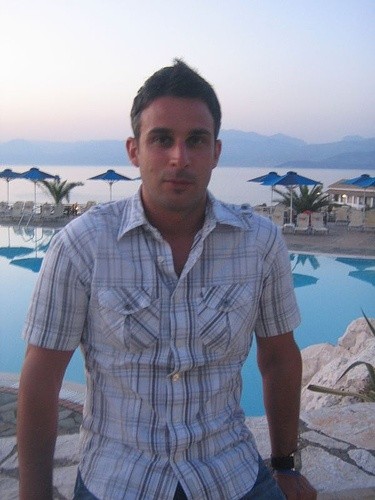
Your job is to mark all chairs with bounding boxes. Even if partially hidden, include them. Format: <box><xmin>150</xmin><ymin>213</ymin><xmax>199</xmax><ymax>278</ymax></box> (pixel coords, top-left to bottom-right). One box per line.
<box><xmin>293</xmin><ymin>205</ymin><xmax>375</xmax><ymax>235</ymax></box>
<box><xmin>0</xmin><ymin>201</ymin><xmax>97</xmax><ymax>223</ymax></box>
<box><xmin>272</xmin><ymin>207</ymin><xmax>285</xmax><ymax>232</ymax></box>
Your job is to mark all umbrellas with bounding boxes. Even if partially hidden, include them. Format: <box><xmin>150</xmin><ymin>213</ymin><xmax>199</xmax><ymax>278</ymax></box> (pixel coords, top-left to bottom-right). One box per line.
<box><xmin>338</xmin><ymin>174</ymin><xmax>375</xmax><ymax>218</ymax></box>
<box><xmin>247</xmin><ymin>172</ymin><xmax>280</xmax><ymax>206</ymax></box>
<box><xmin>0</xmin><ymin>226</ymin><xmax>33</xmax><ymax>260</ymax></box>
<box><xmin>10</xmin><ymin>227</ymin><xmax>43</xmax><ymax>273</ymax></box>
<box><xmin>0</xmin><ymin>168</ymin><xmax>21</xmax><ymax>203</ymax></box>
<box><xmin>86</xmin><ymin>169</ymin><xmax>131</xmax><ymax>202</ymax></box>
<box><xmin>14</xmin><ymin>167</ymin><xmax>56</xmax><ymax>204</ymax></box>
<box><xmin>261</xmin><ymin>171</ymin><xmax>323</xmax><ymax>224</ymax></box>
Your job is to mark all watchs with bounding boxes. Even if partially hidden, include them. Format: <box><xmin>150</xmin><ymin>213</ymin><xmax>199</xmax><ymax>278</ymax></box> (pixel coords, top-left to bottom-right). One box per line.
<box><xmin>271</xmin><ymin>450</ymin><xmax>302</xmax><ymax>472</ymax></box>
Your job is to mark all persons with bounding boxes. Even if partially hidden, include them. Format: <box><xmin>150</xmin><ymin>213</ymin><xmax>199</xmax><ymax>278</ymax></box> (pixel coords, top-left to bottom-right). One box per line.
<box><xmin>17</xmin><ymin>61</ymin><xmax>317</xmax><ymax>500</ymax></box>
<box><xmin>328</xmin><ymin>198</ymin><xmax>337</xmax><ymax>222</ymax></box>
<box><xmin>346</xmin><ymin>205</ymin><xmax>351</xmax><ymax>220</ymax></box>
